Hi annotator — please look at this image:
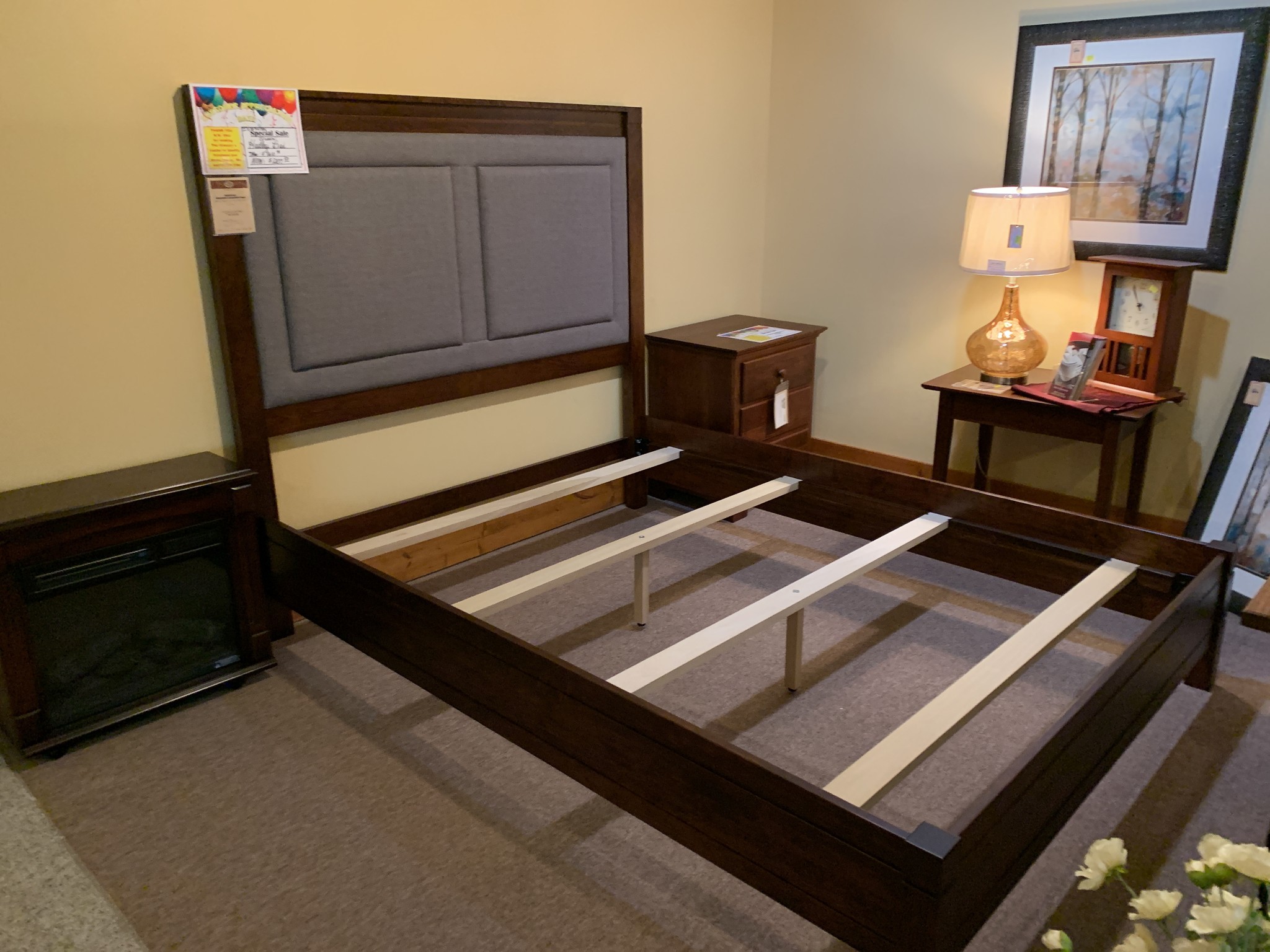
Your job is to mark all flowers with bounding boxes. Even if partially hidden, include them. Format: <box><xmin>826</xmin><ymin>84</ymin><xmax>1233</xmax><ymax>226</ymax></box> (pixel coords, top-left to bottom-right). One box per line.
<box><xmin>1042</xmin><ymin>838</ymin><xmax>1270</xmax><ymax>952</ymax></box>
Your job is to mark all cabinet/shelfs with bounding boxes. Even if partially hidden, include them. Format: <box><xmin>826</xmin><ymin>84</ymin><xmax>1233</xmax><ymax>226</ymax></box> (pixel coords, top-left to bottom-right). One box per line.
<box><xmin>643</xmin><ymin>315</ymin><xmax>827</xmax><ymax>451</ymax></box>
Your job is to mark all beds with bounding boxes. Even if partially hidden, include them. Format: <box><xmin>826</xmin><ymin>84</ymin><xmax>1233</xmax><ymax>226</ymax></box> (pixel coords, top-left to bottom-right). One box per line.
<box><xmin>179</xmin><ymin>81</ymin><xmax>1236</xmax><ymax>952</ymax></box>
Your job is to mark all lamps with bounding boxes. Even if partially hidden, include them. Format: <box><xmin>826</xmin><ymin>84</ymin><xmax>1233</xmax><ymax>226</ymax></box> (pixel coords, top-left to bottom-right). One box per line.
<box><xmin>958</xmin><ymin>185</ymin><xmax>1072</xmax><ymax>385</ymax></box>
<box><xmin>1058</xmin><ymin>181</ymin><xmax>1139</xmax><ymax>221</ymax></box>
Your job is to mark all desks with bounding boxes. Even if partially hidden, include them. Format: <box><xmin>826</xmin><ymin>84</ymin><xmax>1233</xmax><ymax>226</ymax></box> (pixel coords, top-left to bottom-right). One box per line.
<box><xmin>922</xmin><ymin>362</ymin><xmax>1181</xmax><ymax>511</ymax></box>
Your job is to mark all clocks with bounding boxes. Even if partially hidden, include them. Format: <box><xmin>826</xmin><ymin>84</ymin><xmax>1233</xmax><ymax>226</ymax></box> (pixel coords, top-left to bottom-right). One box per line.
<box><xmin>1086</xmin><ymin>255</ymin><xmax>1206</xmax><ymax>399</ymax></box>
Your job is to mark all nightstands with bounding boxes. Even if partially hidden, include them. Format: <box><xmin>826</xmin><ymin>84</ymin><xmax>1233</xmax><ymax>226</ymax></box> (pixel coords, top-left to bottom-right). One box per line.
<box><xmin>0</xmin><ymin>453</ymin><xmax>293</xmax><ymax>760</ymax></box>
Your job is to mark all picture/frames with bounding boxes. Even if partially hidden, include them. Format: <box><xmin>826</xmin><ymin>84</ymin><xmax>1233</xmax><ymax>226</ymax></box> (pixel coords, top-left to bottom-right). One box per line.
<box><xmin>1002</xmin><ymin>7</ymin><xmax>1270</xmax><ymax>273</ymax></box>
<box><xmin>1177</xmin><ymin>356</ymin><xmax>1270</xmax><ymax>616</ymax></box>
